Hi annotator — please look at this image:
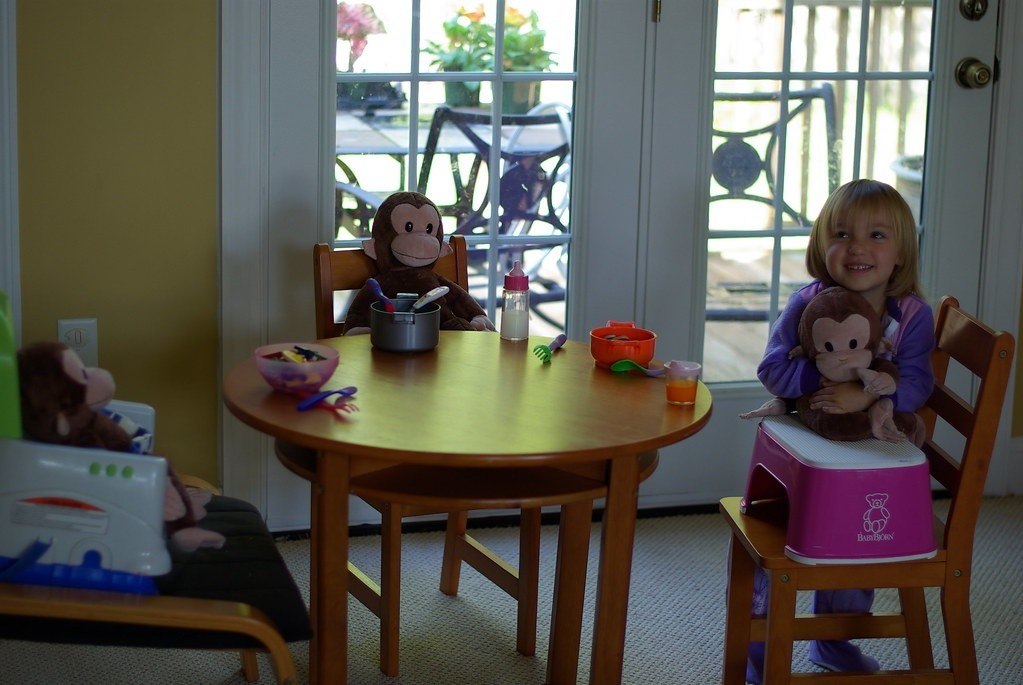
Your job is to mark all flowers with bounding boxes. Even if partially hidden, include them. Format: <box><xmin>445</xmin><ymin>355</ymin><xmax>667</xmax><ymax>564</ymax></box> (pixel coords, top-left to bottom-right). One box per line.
<box><xmin>420</xmin><ymin>2</ymin><xmax>561</xmax><ymax>90</ymax></box>
<box><xmin>337</xmin><ymin>2</ymin><xmax>388</xmax><ymax>73</ymax></box>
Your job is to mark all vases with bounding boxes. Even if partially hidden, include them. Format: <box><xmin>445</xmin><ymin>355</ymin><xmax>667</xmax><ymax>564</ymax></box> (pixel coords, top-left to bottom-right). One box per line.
<box><xmin>500</xmin><ymin>79</ymin><xmax>542</xmax><ymax>114</ymax></box>
<box><xmin>442</xmin><ymin>82</ymin><xmax>479</xmax><ymax>106</ymax></box>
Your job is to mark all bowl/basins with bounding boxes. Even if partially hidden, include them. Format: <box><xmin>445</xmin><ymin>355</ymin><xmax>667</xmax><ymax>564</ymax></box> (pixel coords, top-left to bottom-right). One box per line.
<box><xmin>590</xmin><ymin>320</ymin><xmax>658</xmax><ymax>369</ymax></box>
<box><xmin>254</xmin><ymin>341</ymin><xmax>340</xmax><ymax>393</ymax></box>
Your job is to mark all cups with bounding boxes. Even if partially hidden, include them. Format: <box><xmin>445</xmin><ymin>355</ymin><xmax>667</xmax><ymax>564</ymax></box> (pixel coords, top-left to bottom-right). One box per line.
<box><xmin>664</xmin><ymin>360</ymin><xmax>701</xmax><ymax>406</ymax></box>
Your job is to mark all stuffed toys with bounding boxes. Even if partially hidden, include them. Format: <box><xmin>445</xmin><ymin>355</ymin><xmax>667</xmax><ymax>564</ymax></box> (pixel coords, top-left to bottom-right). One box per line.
<box><xmin>17</xmin><ymin>343</ymin><xmax>225</xmax><ymax>551</ymax></box>
<box><xmin>342</xmin><ymin>191</ymin><xmax>496</xmax><ymax>335</ymax></box>
<box><xmin>740</xmin><ymin>287</ymin><xmax>926</xmax><ymax>448</ymax></box>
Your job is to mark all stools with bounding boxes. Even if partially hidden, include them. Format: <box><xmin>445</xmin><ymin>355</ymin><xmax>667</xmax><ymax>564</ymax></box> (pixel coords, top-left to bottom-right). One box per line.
<box><xmin>740</xmin><ymin>416</ymin><xmax>938</xmax><ymax>563</ymax></box>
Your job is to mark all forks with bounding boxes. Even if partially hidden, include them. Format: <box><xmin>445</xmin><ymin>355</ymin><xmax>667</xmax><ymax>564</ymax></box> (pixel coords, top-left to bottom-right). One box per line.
<box><xmin>534</xmin><ymin>333</ymin><xmax>567</xmax><ymax>362</ymax></box>
<box><xmin>296</xmin><ymin>391</ymin><xmax>359</xmax><ymax>413</ymax></box>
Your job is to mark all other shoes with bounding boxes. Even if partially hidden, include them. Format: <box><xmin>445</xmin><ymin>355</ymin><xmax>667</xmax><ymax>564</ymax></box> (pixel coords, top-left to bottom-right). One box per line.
<box><xmin>809</xmin><ymin>639</ymin><xmax>879</xmax><ymax>673</ymax></box>
<box><xmin>746</xmin><ymin>642</ymin><xmax>765</xmax><ymax>685</ymax></box>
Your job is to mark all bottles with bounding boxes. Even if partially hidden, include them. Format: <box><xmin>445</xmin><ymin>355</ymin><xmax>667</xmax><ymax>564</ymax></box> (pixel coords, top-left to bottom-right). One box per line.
<box><xmin>500</xmin><ymin>261</ymin><xmax>530</xmax><ymax>341</ymax></box>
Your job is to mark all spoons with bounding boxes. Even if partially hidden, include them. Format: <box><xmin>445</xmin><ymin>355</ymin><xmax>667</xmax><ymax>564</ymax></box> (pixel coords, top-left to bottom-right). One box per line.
<box><xmin>610</xmin><ymin>360</ymin><xmax>665</xmax><ymax>377</ymax></box>
<box><xmin>298</xmin><ymin>386</ymin><xmax>356</xmax><ymax>410</ymax></box>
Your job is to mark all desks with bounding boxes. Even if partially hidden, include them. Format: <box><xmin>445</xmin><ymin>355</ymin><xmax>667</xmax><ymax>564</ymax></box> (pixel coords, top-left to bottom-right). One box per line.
<box><xmin>219</xmin><ymin>332</ymin><xmax>713</xmax><ymax>685</ymax></box>
<box><xmin>336</xmin><ymin>107</ymin><xmax>578</xmax><ymax>276</ymax></box>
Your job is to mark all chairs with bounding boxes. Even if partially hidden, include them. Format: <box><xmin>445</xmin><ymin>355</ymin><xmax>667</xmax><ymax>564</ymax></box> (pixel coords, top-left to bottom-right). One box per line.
<box><xmin>333</xmin><ymin>107</ymin><xmax>572</xmax><ymax>323</ymax></box>
<box><xmin>705</xmin><ymin>85</ymin><xmax>838</xmax><ymax>321</ymax></box>
<box><xmin>718</xmin><ymin>295</ymin><xmax>1015</xmax><ymax>685</ymax></box>
<box><xmin>0</xmin><ymin>473</ymin><xmax>297</xmax><ymax>685</ymax></box>
<box><xmin>313</xmin><ymin>236</ymin><xmax>543</xmax><ymax>676</ymax></box>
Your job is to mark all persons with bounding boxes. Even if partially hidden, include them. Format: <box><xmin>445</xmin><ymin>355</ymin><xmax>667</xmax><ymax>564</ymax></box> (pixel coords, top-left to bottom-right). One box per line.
<box><xmin>725</xmin><ymin>179</ymin><xmax>934</xmax><ymax>685</ymax></box>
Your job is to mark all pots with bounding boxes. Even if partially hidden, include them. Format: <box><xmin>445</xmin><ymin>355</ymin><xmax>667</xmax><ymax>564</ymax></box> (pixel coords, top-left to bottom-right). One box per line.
<box><xmin>370</xmin><ymin>293</ymin><xmax>441</xmax><ymax>351</ymax></box>
